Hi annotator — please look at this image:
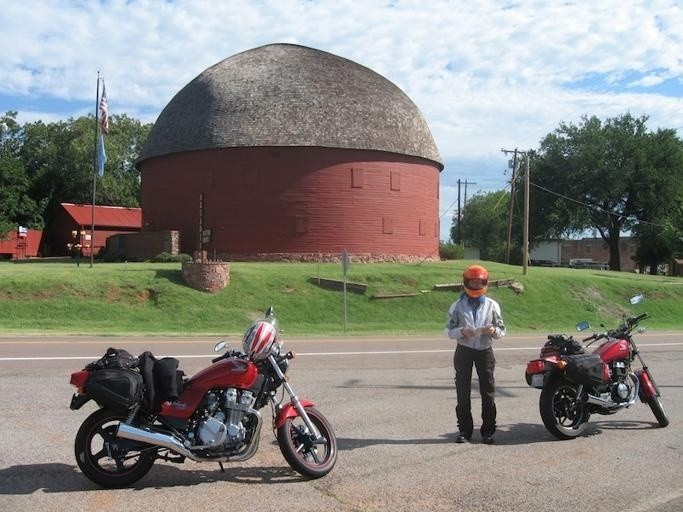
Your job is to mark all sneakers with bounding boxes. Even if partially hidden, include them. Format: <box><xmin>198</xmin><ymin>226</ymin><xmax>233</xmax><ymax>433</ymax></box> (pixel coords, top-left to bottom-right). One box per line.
<box><xmin>456</xmin><ymin>431</ymin><xmax>471</xmax><ymax>443</ymax></box>
<box><xmin>482</xmin><ymin>434</ymin><xmax>494</xmax><ymax>444</ymax></box>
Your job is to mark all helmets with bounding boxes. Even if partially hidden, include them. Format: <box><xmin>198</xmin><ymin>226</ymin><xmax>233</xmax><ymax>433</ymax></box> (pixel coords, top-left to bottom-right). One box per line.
<box><xmin>241</xmin><ymin>316</ymin><xmax>279</xmax><ymax>362</ymax></box>
<box><xmin>462</xmin><ymin>264</ymin><xmax>489</xmax><ymax>299</ymax></box>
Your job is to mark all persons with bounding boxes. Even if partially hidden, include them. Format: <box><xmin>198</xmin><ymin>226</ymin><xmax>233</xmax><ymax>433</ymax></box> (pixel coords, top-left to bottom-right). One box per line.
<box><xmin>445</xmin><ymin>263</ymin><xmax>506</xmax><ymax>445</ymax></box>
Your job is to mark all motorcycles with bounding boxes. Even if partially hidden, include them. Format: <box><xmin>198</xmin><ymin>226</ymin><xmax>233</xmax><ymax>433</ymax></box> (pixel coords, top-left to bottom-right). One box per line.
<box><xmin>521</xmin><ymin>294</ymin><xmax>670</xmax><ymax>439</ymax></box>
<box><xmin>69</xmin><ymin>304</ymin><xmax>338</xmax><ymax>487</ymax></box>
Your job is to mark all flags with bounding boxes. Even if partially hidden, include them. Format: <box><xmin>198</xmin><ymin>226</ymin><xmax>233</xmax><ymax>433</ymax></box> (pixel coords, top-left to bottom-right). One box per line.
<box><xmin>97</xmin><ymin>77</ymin><xmax>109</xmax><ymax>137</ymax></box>
<box><xmin>95</xmin><ymin>128</ymin><xmax>108</xmax><ymax>180</ymax></box>
